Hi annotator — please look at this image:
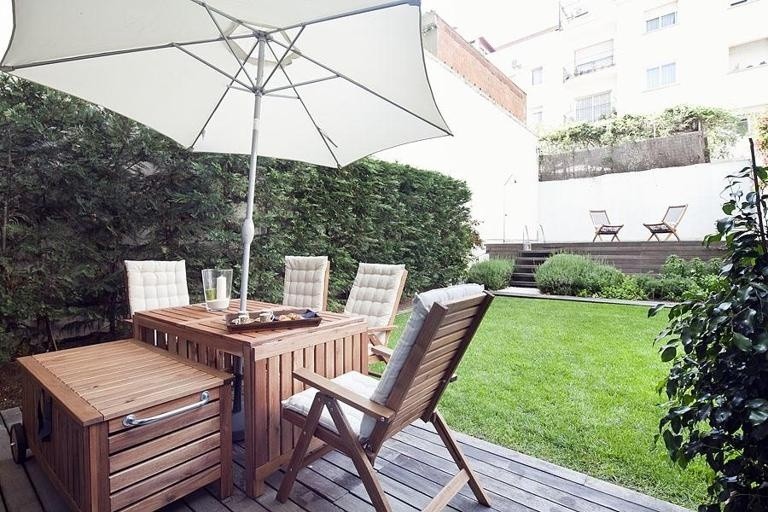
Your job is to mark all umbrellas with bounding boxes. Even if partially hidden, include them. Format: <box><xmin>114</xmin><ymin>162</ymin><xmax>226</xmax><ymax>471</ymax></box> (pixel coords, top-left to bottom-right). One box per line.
<box><xmin>0</xmin><ymin>0</ymin><xmax>455</xmax><ymax>311</ymax></box>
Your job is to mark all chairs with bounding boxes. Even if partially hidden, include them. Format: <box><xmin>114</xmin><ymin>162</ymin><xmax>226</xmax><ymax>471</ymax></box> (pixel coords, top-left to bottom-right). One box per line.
<box><xmin>273</xmin><ymin>281</ymin><xmax>495</xmax><ymax>510</ymax></box>
<box><xmin>344</xmin><ymin>262</ymin><xmax>410</xmax><ymax>371</ymax></box>
<box><xmin>279</xmin><ymin>254</ymin><xmax>330</xmax><ymax>314</ymax></box>
<box><xmin>124</xmin><ymin>256</ymin><xmax>192</xmax><ymax>347</ymax></box>
<box><xmin>590</xmin><ymin>206</ymin><xmax>686</xmax><ymax>242</ymax></box>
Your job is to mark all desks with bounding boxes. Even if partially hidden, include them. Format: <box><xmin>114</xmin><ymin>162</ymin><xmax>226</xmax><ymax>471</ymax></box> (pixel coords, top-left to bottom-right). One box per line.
<box><xmin>11</xmin><ymin>340</ymin><xmax>235</xmax><ymax>510</ymax></box>
<box><xmin>136</xmin><ymin>295</ymin><xmax>370</xmax><ymax>499</ymax></box>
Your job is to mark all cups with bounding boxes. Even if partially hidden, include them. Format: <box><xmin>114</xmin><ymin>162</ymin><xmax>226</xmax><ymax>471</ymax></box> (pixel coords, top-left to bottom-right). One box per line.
<box><xmin>232</xmin><ymin>309</ymin><xmax>276</xmax><ymax>325</ymax></box>
<box><xmin>201</xmin><ymin>267</ymin><xmax>235</xmax><ymax>313</ymax></box>
